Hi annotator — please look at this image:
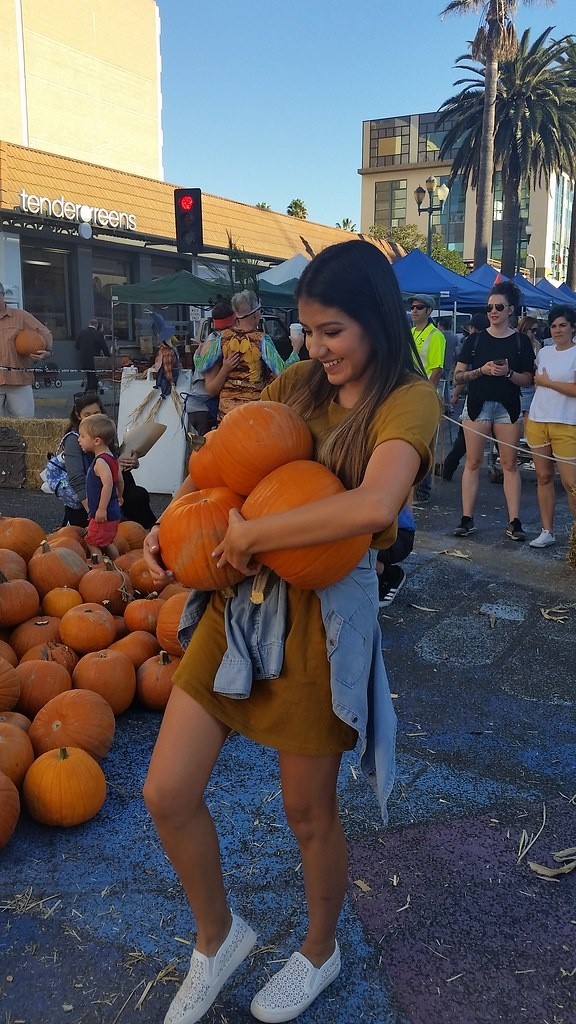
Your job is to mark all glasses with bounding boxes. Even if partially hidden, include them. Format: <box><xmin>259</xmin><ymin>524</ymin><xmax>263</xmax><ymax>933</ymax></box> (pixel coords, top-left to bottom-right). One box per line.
<box><xmin>410</xmin><ymin>303</ymin><xmax>429</xmax><ymax>310</ymax></box>
<box><xmin>527</xmin><ymin>328</ymin><xmax>538</xmax><ymax>333</ymax></box>
<box><xmin>486</xmin><ymin>303</ymin><xmax>511</xmax><ymax>312</ymax></box>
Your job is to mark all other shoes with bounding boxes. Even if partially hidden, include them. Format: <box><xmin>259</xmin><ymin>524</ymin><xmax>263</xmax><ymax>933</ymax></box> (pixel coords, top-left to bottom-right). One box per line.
<box><xmin>444</xmin><ymin>409</ymin><xmax>454</xmax><ymax>417</ymax></box>
<box><xmin>163</xmin><ymin>915</ymin><xmax>255</xmax><ymax>1024</ymax></box>
<box><xmin>412</xmin><ymin>491</ymin><xmax>430</xmax><ymax>505</ymax></box>
<box><xmin>251</xmin><ymin>939</ymin><xmax>341</xmax><ymax>1023</ymax></box>
<box><xmin>450</xmin><ymin>393</ymin><xmax>461</xmax><ymax>399</ymax></box>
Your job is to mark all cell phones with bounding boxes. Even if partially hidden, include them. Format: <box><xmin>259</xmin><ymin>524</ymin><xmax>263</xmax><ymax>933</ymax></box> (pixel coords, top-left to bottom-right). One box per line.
<box><xmin>493</xmin><ymin>359</ymin><xmax>505</xmax><ymax>367</ymax></box>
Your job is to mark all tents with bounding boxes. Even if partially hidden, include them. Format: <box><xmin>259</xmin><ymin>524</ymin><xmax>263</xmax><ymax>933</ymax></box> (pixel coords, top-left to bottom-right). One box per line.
<box><xmin>111</xmin><ymin>254</ymin><xmax>312</xmax><ymax>419</ymax></box>
<box><xmin>389</xmin><ymin>248</ymin><xmax>576</xmax><ymax>337</ymax></box>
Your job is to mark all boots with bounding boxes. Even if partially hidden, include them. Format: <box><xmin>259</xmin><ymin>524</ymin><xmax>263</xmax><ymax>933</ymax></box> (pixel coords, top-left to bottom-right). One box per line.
<box><xmin>432</xmin><ymin>453</ymin><xmax>460</xmax><ymax>481</ymax></box>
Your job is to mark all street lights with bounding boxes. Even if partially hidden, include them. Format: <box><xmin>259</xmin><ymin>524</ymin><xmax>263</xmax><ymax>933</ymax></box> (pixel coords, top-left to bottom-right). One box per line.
<box><xmin>527</xmin><ymin>254</ymin><xmax>536</xmax><ymax>286</ymax></box>
<box><xmin>516</xmin><ymin>207</ymin><xmax>533</xmax><ymax>272</ymax></box>
<box><xmin>412</xmin><ymin>174</ymin><xmax>450</xmax><ymax>256</ymax></box>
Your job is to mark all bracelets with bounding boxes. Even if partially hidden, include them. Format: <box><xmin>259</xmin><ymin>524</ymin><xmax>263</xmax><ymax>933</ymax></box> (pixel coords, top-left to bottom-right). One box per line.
<box><xmin>47</xmin><ymin>350</ymin><xmax>53</xmax><ymax>358</ymax></box>
<box><xmin>149</xmin><ymin>523</ymin><xmax>161</xmax><ymax>531</ymax></box>
<box><xmin>505</xmin><ymin>369</ymin><xmax>514</xmax><ymax>378</ymax></box>
<box><xmin>480</xmin><ymin>367</ymin><xmax>484</xmax><ymax>376</ymax></box>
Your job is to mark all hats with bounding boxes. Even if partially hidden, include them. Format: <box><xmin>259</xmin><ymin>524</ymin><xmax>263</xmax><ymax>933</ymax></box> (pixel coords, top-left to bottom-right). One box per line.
<box><xmin>212</xmin><ymin>303</ymin><xmax>236</xmax><ymax>329</ymax></box>
<box><xmin>231</xmin><ymin>289</ymin><xmax>261</xmax><ymax>318</ymax></box>
<box><xmin>407</xmin><ymin>294</ymin><xmax>435</xmax><ymax>310</ymax></box>
<box><xmin>464</xmin><ymin>314</ymin><xmax>491</xmax><ymax>332</ymax></box>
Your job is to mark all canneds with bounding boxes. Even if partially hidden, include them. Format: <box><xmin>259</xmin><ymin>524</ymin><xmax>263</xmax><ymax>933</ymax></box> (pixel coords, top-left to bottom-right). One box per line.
<box><xmin>0</xmin><ymin>516</ymin><xmax>192</xmax><ymax>852</ymax></box>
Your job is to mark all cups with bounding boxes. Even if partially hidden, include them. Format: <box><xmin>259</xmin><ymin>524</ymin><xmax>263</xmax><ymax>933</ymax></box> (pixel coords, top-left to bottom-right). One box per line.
<box><xmin>290</xmin><ymin>323</ymin><xmax>304</xmax><ymax>346</ymax></box>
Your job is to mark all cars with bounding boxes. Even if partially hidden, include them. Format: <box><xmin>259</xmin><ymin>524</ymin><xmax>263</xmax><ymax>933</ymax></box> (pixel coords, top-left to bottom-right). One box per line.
<box><xmin>191</xmin><ymin>315</ymin><xmax>292</xmax><ymax>366</ymax></box>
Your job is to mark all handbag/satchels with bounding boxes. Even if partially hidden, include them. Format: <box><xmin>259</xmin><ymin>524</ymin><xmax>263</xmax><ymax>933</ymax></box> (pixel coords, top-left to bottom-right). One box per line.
<box><xmin>45</xmin><ymin>431</ymin><xmax>90</xmax><ymax>509</ymax></box>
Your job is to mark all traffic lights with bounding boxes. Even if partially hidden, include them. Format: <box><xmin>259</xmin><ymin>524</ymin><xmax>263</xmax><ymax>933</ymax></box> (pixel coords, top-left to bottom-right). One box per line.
<box><xmin>174</xmin><ymin>188</ymin><xmax>203</xmax><ymax>254</ymax></box>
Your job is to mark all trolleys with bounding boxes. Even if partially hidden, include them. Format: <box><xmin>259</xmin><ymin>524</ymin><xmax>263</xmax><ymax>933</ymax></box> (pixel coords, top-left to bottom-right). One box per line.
<box><xmin>93</xmin><ymin>353</ymin><xmax>128</xmax><ymax>395</ymax></box>
<box><xmin>32</xmin><ymin>364</ymin><xmax>62</xmax><ymax>388</ymax></box>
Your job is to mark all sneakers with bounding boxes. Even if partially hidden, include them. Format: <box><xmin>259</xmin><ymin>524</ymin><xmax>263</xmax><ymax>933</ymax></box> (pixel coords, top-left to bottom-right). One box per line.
<box><xmin>529</xmin><ymin>527</ymin><xmax>556</xmax><ymax>547</ymax></box>
<box><xmin>378</xmin><ymin>565</ymin><xmax>406</xmax><ymax>607</ymax></box>
<box><xmin>506</xmin><ymin>517</ymin><xmax>527</xmax><ymax>540</ymax></box>
<box><xmin>453</xmin><ymin>515</ymin><xmax>475</xmax><ymax>536</ymax></box>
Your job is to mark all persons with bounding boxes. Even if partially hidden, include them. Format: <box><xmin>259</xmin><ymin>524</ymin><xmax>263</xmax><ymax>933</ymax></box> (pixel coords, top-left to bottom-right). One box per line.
<box><xmin>407</xmin><ymin>281</ymin><xmax>576</xmax><ymax>549</ymax></box>
<box><xmin>144</xmin><ymin>240</ymin><xmax>445</xmax><ymax>1024</ymax></box>
<box><xmin>63</xmin><ymin>289</ymin><xmax>304</xmax><ymax>562</ymax></box>
<box><xmin>0</xmin><ymin>283</ymin><xmax>55</xmax><ymax>419</ymax></box>
<box><xmin>376</xmin><ymin>494</ymin><xmax>416</xmax><ymax>608</ymax></box>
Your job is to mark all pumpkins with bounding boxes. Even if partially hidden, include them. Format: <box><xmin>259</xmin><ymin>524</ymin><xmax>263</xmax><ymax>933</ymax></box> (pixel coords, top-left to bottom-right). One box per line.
<box><xmin>15</xmin><ymin>328</ymin><xmax>47</xmax><ymax>356</ymax></box>
<box><xmin>159</xmin><ymin>400</ymin><xmax>372</xmax><ymax>605</ymax></box>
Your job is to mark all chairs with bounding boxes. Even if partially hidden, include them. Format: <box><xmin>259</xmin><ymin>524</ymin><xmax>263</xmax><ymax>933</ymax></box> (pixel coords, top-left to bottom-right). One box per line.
<box><xmin>94</xmin><ymin>356</ymin><xmax>125</xmax><ymax>421</ymax></box>
<box><xmin>437</xmin><ymin>380</ymin><xmax>452</xmax><ymax>447</ymax></box>
